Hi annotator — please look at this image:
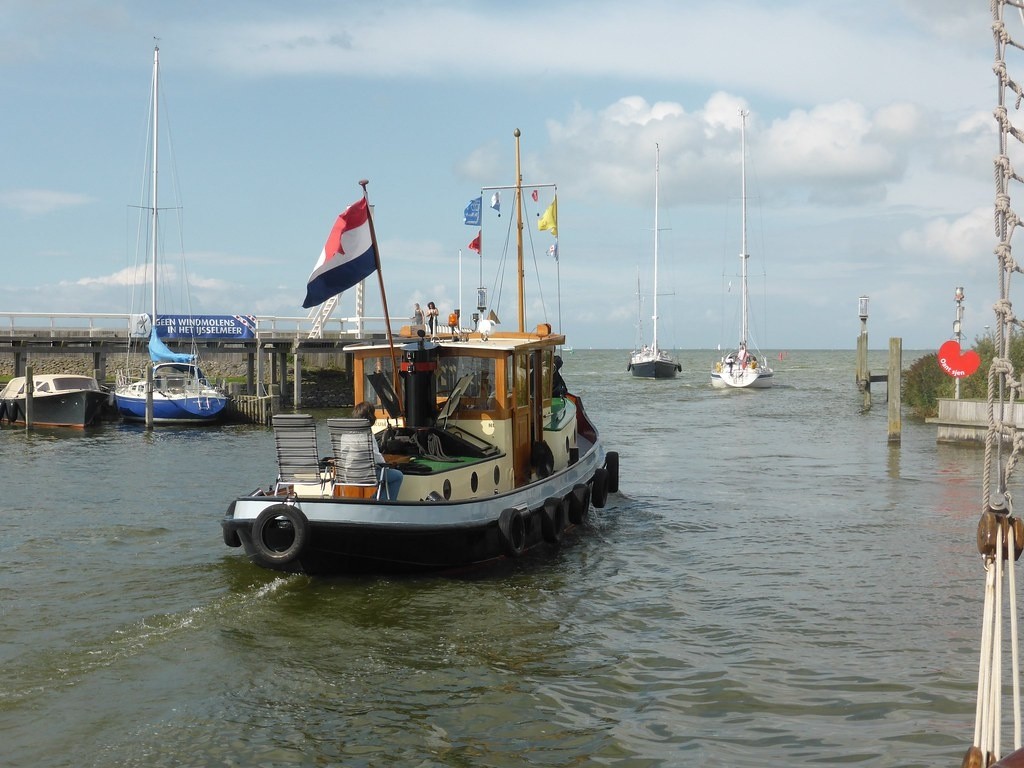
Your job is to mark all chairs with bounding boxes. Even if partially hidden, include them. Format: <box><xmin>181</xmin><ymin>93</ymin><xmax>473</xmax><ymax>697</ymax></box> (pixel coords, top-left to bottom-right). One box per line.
<box><xmin>327</xmin><ymin>418</ymin><xmax>392</xmax><ymax>501</ymax></box>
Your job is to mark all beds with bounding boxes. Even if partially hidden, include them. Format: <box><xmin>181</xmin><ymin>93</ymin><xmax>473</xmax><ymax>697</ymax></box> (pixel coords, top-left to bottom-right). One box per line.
<box><xmin>272</xmin><ymin>414</ymin><xmax>335</xmax><ymax>499</ymax></box>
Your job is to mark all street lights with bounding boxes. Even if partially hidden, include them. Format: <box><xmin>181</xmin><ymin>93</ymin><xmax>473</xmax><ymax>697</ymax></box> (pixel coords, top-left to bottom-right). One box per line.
<box><xmin>954</xmin><ymin>286</ymin><xmax>964</xmax><ymax>400</ymax></box>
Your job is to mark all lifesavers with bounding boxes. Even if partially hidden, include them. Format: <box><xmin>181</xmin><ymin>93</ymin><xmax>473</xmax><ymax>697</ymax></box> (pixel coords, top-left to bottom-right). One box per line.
<box><xmin>497</xmin><ymin>508</ymin><xmax>525</xmax><ymax>557</ymax></box>
<box><xmin>591</xmin><ymin>469</ymin><xmax>610</xmax><ymax>508</ymax></box>
<box><xmin>221</xmin><ymin>498</ymin><xmax>243</xmax><ymax>548</ymax></box>
<box><xmin>540</xmin><ymin>497</ymin><xmax>565</xmax><ymax>543</ymax></box>
<box><xmin>716</xmin><ymin>362</ymin><xmax>720</xmax><ymax>372</ymax></box>
<box><xmin>569</xmin><ymin>484</ymin><xmax>590</xmax><ymax>524</ymax></box>
<box><xmin>252</xmin><ymin>504</ymin><xmax>310</xmax><ymax>563</ymax></box>
<box><xmin>605</xmin><ymin>451</ymin><xmax>619</xmax><ymax>493</ymax></box>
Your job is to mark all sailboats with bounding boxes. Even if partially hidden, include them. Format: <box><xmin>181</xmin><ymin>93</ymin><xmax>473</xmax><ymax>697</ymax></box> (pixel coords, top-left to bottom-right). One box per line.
<box><xmin>627</xmin><ymin>140</ymin><xmax>683</xmax><ymax>381</ymax></box>
<box><xmin>111</xmin><ymin>47</ymin><xmax>227</xmax><ymax>425</ymax></box>
<box><xmin>710</xmin><ymin>109</ymin><xmax>776</xmax><ymax>388</ymax></box>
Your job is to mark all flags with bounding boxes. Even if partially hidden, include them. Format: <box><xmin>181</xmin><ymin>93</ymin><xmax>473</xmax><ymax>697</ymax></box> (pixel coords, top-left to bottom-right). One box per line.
<box><xmin>468</xmin><ymin>230</ymin><xmax>481</xmax><ymax>254</ymax></box>
<box><xmin>489</xmin><ymin>192</ymin><xmax>500</xmax><ymax>211</ymax></box>
<box><xmin>302</xmin><ymin>197</ymin><xmax>381</xmax><ymax>308</ymax></box>
<box><xmin>538</xmin><ymin>200</ymin><xmax>557</xmax><ymax>237</ymax></box>
<box><xmin>546</xmin><ymin>241</ymin><xmax>558</xmax><ymax>264</ymax></box>
<box><xmin>464</xmin><ymin>196</ymin><xmax>482</xmax><ymax>226</ymax></box>
<box><xmin>532</xmin><ymin>190</ymin><xmax>538</xmax><ymax>201</ymax></box>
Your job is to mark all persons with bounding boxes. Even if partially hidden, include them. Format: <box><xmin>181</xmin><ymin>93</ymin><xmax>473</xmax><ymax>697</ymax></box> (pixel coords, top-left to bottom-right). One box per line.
<box><xmin>716</xmin><ymin>362</ymin><xmax>721</xmax><ymax>372</ymax></box>
<box><xmin>737</xmin><ymin>347</ymin><xmax>750</xmax><ymax>370</ymax></box>
<box><xmin>520</xmin><ymin>344</ymin><xmax>568</xmax><ymax>399</ymax></box>
<box><xmin>751</xmin><ymin>357</ymin><xmax>757</xmax><ymax>369</ymax></box>
<box><xmin>411</xmin><ymin>303</ymin><xmax>425</xmax><ymax>325</ymax></box>
<box><xmin>340</xmin><ymin>401</ymin><xmax>403</xmax><ymax>500</ymax></box>
<box><xmin>426</xmin><ymin>302</ymin><xmax>439</xmax><ymax>334</ymax></box>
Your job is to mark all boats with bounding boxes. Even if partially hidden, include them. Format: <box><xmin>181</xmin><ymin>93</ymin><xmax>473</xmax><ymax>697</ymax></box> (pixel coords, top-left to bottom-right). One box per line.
<box><xmin>219</xmin><ymin>128</ymin><xmax>618</xmax><ymax>572</ymax></box>
<box><xmin>0</xmin><ymin>373</ymin><xmax>108</xmax><ymax>429</ymax></box>
